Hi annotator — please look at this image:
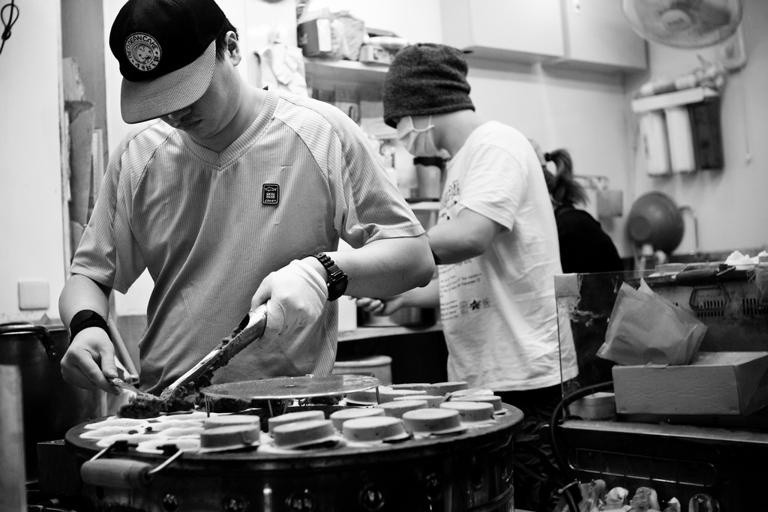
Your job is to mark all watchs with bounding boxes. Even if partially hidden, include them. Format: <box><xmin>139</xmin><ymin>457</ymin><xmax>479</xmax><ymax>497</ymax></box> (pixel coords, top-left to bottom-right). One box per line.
<box><xmin>301</xmin><ymin>252</ymin><xmax>348</xmax><ymax>301</ymax></box>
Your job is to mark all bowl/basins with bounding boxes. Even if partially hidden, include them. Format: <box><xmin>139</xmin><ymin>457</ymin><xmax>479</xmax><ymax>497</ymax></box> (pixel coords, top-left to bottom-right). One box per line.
<box><xmin>629</xmin><ymin>192</ymin><xmax>683</xmax><ymax>254</ymax></box>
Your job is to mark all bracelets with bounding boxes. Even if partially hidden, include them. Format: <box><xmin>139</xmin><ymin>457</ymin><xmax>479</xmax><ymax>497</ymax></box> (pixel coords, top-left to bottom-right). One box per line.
<box><xmin>66</xmin><ymin>309</ymin><xmax>107</xmax><ymax>341</ymax></box>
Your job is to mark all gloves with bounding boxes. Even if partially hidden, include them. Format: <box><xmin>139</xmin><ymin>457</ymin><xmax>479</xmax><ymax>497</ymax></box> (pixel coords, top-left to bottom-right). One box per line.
<box><xmin>246</xmin><ymin>257</ymin><xmax>331</xmax><ymax>356</ymax></box>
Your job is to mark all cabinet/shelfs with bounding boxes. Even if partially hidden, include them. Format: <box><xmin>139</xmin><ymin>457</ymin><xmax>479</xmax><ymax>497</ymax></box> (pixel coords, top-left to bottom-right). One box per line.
<box><xmin>549</xmin><ymin>1</ymin><xmax>647</xmax><ymax>73</ymax></box>
<box><xmin>447</xmin><ymin>0</ymin><xmax>561</xmax><ymax>64</ymax></box>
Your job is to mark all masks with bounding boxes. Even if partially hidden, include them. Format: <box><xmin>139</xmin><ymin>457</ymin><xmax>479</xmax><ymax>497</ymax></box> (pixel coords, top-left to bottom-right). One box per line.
<box><xmin>395</xmin><ymin>112</ymin><xmax>441</xmax><ymax>158</ymax></box>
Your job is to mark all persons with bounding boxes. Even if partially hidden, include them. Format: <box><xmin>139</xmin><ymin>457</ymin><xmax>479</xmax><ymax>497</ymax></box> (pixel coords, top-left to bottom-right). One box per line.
<box><xmin>537</xmin><ymin>148</ymin><xmax>624</xmax><ymax>383</ymax></box>
<box><xmin>61</xmin><ymin>1</ymin><xmax>434</xmax><ymax>396</ymax></box>
<box><xmin>358</xmin><ymin>42</ymin><xmax>579</xmax><ymax>421</ymax></box>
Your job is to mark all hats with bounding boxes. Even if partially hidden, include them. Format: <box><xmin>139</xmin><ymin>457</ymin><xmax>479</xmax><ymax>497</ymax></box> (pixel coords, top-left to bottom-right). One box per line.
<box><xmin>381</xmin><ymin>42</ymin><xmax>477</xmax><ymax>129</ymax></box>
<box><xmin>525</xmin><ymin>136</ymin><xmax>548</xmax><ymax>169</ymax></box>
<box><xmin>108</xmin><ymin>0</ymin><xmax>227</xmax><ymax>126</ymax></box>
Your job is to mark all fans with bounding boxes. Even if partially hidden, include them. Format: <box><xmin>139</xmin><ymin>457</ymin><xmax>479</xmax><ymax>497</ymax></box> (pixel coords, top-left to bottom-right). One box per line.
<box><xmin>620</xmin><ymin>0</ymin><xmax>751</xmax><ymax>72</ymax></box>
